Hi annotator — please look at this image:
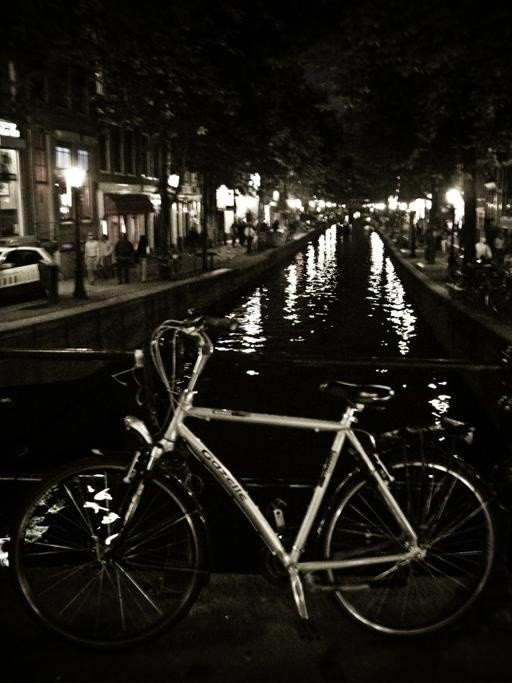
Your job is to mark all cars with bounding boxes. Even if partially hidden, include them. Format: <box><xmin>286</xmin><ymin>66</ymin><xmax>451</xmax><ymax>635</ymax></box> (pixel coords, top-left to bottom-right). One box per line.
<box><xmin>2</xmin><ymin>241</ymin><xmax>58</xmax><ymax>312</ymax></box>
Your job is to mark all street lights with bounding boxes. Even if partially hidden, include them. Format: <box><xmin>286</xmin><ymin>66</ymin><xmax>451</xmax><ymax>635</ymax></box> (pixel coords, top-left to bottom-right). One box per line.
<box><xmin>64</xmin><ymin>164</ymin><xmax>94</xmax><ymax>303</ymax></box>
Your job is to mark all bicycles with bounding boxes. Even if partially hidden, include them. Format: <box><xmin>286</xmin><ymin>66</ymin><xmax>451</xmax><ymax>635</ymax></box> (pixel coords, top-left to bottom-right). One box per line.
<box><xmin>9</xmin><ymin>311</ymin><xmax>495</xmax><ymax>649</ymax></box>
<box><xmin>443</xmin><ymin>255</ymin><xmax>512</xmax><ymax>321</ymax></box>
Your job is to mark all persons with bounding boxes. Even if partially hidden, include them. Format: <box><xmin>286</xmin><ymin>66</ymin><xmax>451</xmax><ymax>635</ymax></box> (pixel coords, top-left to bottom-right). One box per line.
<box><xmin>84</xmin><ymin>203</ymin><xmax>342</xmax><ymax>285</ymax></box>
<box><xmin>373</xmin><ymin>204</ymin><xmax>506</xmax><ymax>284</ymax></box>
